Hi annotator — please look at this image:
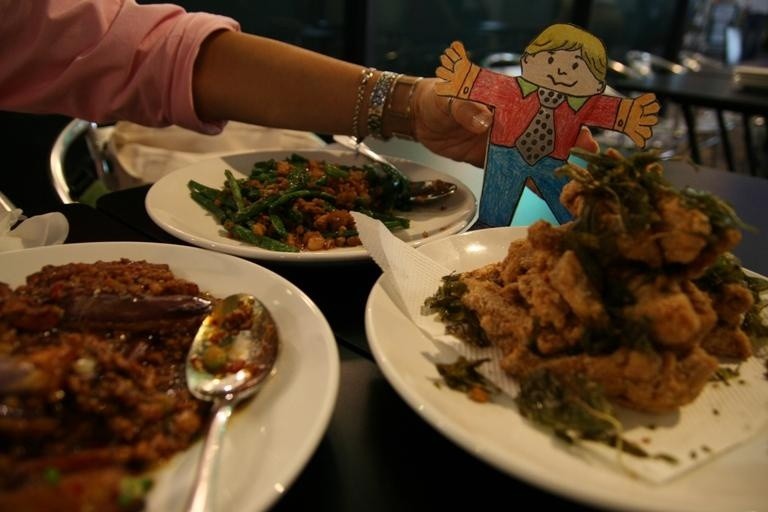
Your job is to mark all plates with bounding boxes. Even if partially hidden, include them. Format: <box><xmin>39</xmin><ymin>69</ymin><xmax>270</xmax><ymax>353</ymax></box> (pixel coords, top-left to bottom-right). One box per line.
<box><xmin>0</xmin><ymin>239</ymin><xmax>343</xmax><ymax>512</ymax></box>
<box><xmin>142</xmin><ymin>146</ymin><xmax>481</xmax><ymax>263</ymax></box>
<box><xmin>359</xmin><ymin>224</ymin><xmax>768</xmax><ymax>512</ymax></box>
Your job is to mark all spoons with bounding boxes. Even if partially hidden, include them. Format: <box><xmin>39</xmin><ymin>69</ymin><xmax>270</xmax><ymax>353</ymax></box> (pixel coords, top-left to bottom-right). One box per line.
<box><xmin>183</xmin><ymin>289</ymin><xmax>284</xmax><ymax>512</ymax></box>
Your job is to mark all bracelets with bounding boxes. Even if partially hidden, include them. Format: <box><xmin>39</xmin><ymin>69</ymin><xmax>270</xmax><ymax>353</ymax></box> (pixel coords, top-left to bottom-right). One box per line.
<box><xmin>349</xmin><ymin>66</ymin><xmax>425</xmax><ymax>145</ymax></box>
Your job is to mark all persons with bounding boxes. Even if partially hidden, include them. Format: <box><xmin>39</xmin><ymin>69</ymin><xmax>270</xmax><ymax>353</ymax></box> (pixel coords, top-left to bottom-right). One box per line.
<box><xmin>0</xmin><ymin>1</ymin><xmax>603</xmax><ymax>212</ymax></box>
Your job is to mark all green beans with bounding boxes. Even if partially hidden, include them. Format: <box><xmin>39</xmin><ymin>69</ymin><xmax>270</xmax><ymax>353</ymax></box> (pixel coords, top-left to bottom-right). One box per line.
<box><xmin>187</xmin><ymin>163</ymin><xmax>409</xmax><ymax>253</ymax></box>
